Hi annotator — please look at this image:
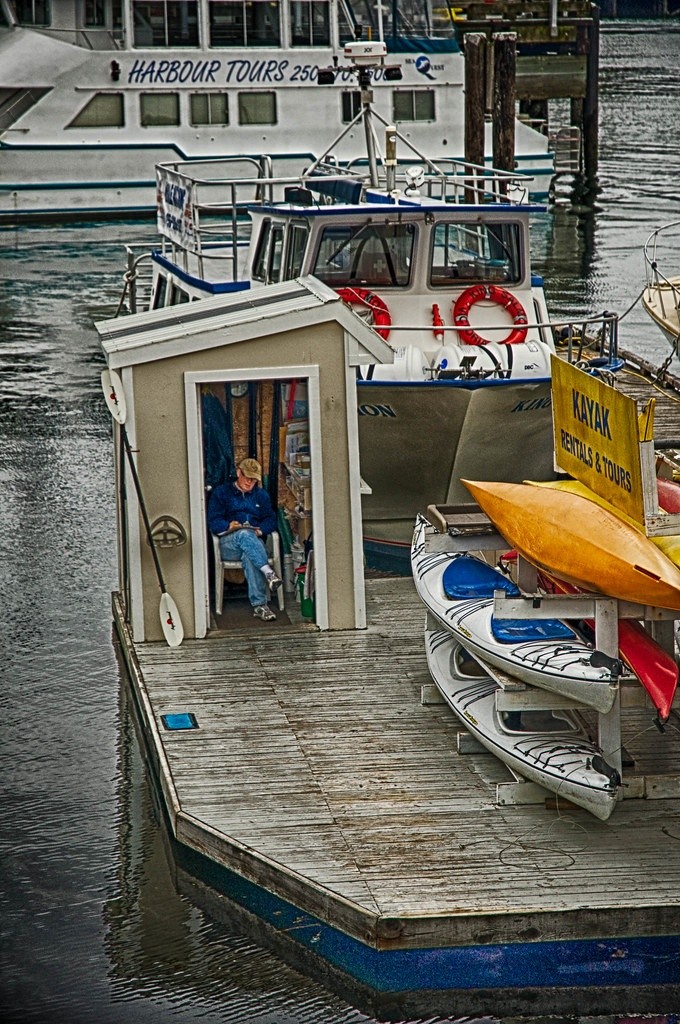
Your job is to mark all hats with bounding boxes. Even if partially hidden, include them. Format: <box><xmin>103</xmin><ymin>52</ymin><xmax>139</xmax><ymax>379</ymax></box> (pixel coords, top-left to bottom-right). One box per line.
<box><xmin>239</xmin><ymin>458</ymin><xmax>262</xmax><ymax>482</ymax></box>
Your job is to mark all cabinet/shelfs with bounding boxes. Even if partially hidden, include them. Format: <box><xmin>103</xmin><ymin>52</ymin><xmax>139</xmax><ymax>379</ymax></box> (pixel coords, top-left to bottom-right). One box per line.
<box><xmin>280</xmin><ymin>461</ymin><xmax>312</xmax><ymax>553</ymax></box>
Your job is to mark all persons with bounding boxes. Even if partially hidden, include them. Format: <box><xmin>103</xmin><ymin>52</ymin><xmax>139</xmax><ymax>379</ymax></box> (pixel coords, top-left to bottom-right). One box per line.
<box><xmin>208</xmin><ymin>458</ymin><xmax>284</xmax><ymax>621</ymax></box>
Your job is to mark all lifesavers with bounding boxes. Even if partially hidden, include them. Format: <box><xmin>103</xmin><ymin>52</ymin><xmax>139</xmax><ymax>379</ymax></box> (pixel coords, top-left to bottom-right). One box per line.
<box><xmin>453</xmin><ymin>282</ymin><xmax>530</xmax><ymax>349</ymax></box>
<box><xmin>336</xmin><ymin>286</ymin><xmax>392</xmax><ymax>340</ymax></box>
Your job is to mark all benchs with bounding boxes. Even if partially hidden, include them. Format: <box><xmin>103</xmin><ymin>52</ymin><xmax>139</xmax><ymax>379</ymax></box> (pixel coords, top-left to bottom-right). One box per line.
<box><xmin>290</xmin><ymin>167</ymin><xmax>362</xmax><ymax>269</ymax></box>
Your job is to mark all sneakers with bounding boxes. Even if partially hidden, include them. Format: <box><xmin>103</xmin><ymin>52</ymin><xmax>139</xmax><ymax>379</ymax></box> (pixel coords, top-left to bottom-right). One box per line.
<box><xmin>265</xmin><ymin>571</ymin><xmax>284</xmax><ymax>592</ymax></box>
<box><xmin>252</xmin><ymin>605</ymin><xmax>277</xmax><ymax>622</ymax></box>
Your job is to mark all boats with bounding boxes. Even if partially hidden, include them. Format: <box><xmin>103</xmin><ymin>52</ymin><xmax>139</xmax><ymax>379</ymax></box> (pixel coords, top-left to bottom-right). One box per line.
<box><xmin>85</xmin><ymin>155</ymin><xmax>680</xmax><ymax>1024</ymax></box>
<box><xmin>0</xmin><ymin>0</ymin><xmax>558</xmax><ymax>215</ymax></box>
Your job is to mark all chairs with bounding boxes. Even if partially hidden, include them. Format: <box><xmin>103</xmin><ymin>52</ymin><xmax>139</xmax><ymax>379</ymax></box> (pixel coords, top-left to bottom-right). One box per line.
<box><xmin>208</xmin><ymin>527</ymin><xmax>285</xmax><ymax>615</ymax></box>
